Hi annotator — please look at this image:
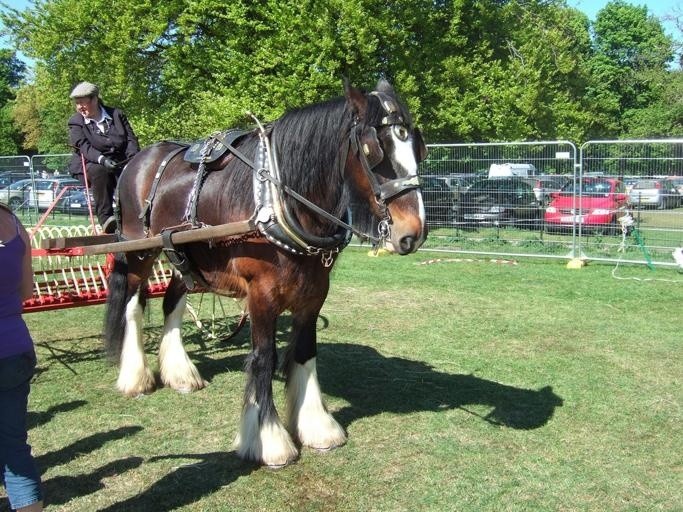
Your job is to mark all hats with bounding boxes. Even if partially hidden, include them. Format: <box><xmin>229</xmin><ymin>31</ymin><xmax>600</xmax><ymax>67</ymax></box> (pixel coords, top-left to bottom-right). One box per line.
<box><xmin>69</xmin><ymin>81</ymin><xmax>100</xmax><ymax>100</ymax></box>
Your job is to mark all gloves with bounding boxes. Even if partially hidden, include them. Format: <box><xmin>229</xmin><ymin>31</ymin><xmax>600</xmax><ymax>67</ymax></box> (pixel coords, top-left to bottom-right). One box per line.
<box><xmin>100</xmin><ymin>156</ymin><xmax>123</xmax><ymax>173</ymax></box>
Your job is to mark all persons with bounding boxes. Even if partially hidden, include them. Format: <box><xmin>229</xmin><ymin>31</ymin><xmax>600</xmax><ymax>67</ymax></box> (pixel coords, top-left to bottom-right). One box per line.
<box><xmin>67</xmin><ymin>81</ymin><xmax>140</xmax><ymax>235</ymax></box>
<box><xmin>0</xmin><ymin>201</ymin><xmax>43</xmax><ymax>512</ymax></box>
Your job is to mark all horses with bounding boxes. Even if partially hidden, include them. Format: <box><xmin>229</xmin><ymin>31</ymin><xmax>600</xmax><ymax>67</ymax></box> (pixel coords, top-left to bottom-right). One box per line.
<box><xmin>102</xmin><ymin>76</ymin><xmax>430</xmax><ymax>467</ymax></box>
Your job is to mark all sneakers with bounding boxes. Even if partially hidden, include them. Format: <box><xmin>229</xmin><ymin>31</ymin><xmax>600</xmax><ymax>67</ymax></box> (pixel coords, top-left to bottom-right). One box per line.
<box><xmin>102</xmin><ymin>215</ymin><xmax>118</xmax><ymax>233</ymax></box>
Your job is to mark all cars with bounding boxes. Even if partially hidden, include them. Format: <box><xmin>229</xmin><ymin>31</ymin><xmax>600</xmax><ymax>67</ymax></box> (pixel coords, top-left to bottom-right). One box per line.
<box><xmin>0</xmin><ymin>168</ymin><xmax>96</xmax><ymax>217</ymax></box>
<box><xmin>414</xmin><ymin>163</ymin><xmax>683</xmax><ymax>236</ymax></box>
